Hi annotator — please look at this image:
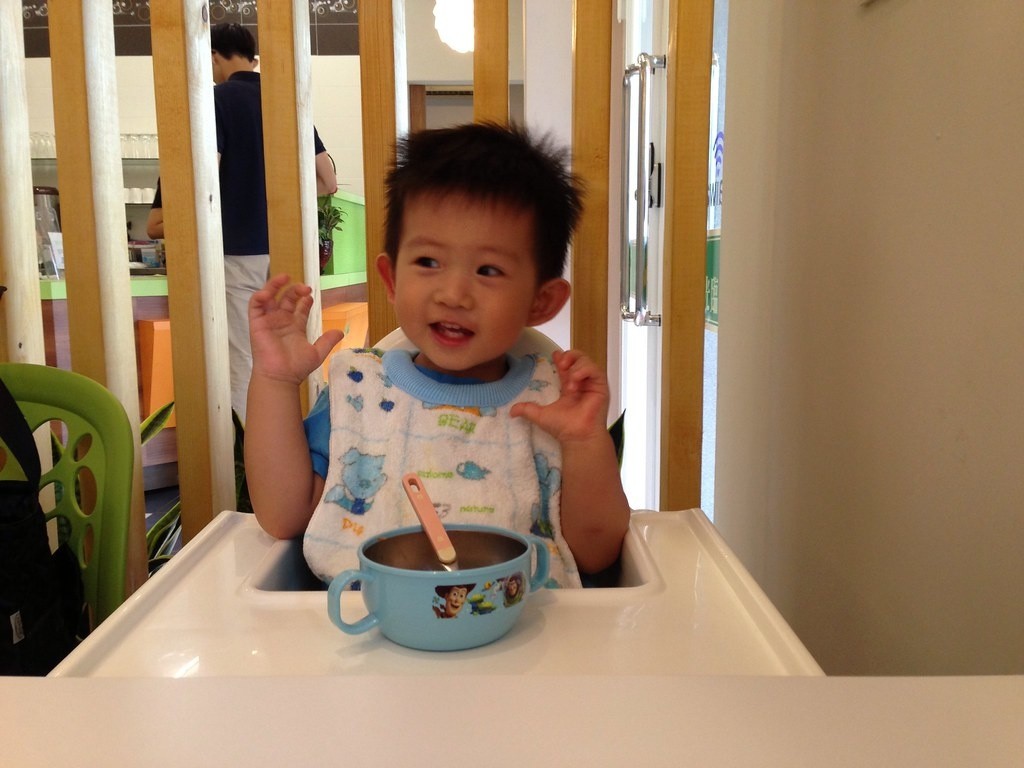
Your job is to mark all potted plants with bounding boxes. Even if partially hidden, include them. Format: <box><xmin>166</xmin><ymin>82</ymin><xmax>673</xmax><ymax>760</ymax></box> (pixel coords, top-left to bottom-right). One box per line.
<box><xmin>318</xmin><ymin>204</ymin><xmax>348</xmax><ymax>276</ymax></box>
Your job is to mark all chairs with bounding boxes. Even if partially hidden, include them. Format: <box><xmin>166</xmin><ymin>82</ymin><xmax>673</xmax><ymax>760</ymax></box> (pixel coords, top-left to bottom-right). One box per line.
<box><xmin>0</xmin><ymin>362</ymin><xmax>134</xmax><ymax>638</ymax></box>
<box><xmin>47</xmin><ymin>326</ymin><xmax>829</xmax><ymax>677</ymax></box>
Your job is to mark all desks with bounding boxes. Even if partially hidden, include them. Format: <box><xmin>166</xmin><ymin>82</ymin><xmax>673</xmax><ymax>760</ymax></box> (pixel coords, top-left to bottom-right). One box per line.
<box><xmin>0</xmin><ymin>674</ymin><xmax>1024</xmax><ymax>768</ymax></box>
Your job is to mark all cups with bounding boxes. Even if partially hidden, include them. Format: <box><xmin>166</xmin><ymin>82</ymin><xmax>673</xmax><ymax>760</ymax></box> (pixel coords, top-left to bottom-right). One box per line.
<box><xmin>29</xmin><ymin>132</ymin><xmax>56</xmax><ymax>159</ymax></box>
<box><xmin>120</xmin><ymin>134</ymin><xmax>158</xmax><ymax>158</ymax></box>
<box><xmin>125</xmin><ymin>187</ymin><xmax>155</xmax><ymax>204</ymax></box>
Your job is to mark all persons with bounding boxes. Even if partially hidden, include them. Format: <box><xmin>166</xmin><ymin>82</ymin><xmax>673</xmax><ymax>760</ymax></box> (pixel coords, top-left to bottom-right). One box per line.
<box><xmin>145</xmin><ymin>22</ymin><xmax>339</xmax><ymax>451</ymax></box>
<box><xmin>243</xmin><ymin>121</ymin><xmax>629</xmax><ymax>587</ymax></box>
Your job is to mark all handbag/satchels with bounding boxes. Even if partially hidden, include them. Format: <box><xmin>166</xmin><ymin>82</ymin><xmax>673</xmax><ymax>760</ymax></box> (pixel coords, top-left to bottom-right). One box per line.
<box><xmin>0</xmin><ymin>375</ymin><xmax>90</xmax><ymax>676</ymax></box>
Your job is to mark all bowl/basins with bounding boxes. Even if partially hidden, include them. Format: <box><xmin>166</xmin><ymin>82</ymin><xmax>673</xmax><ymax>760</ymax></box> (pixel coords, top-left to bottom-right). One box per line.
<box><xmin>329</xmin><ymin>523</ymin><xmax>548</xmax><ymax>652</ymax></box>
